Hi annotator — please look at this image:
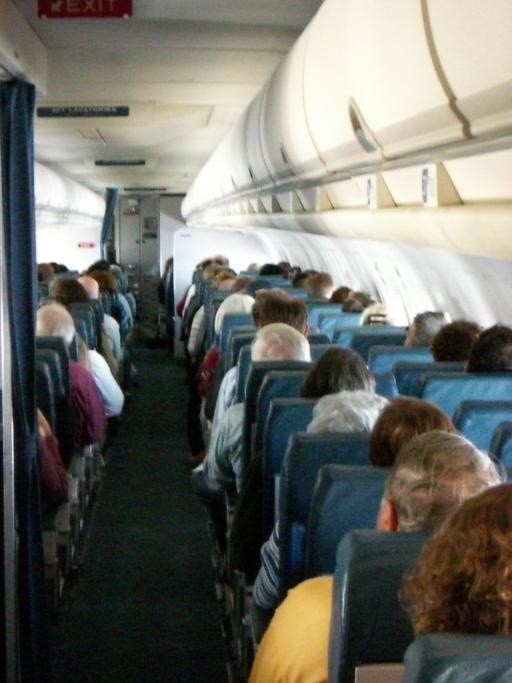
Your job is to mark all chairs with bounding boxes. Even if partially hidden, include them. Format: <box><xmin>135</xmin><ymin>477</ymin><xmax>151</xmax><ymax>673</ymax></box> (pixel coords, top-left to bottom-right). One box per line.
<box><xmin>164</xmin><ymin>253</ymin><xmax>512</xmax><ymax>681</ymax></box>
<box><xmin>32</xmin><ymin>261</ymin><xmax>140</xmax><ymax>617</ymax></box>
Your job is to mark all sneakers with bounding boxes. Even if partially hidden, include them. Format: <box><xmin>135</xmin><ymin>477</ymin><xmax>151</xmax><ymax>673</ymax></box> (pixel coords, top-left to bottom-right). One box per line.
<box><xmin>188</xmin><ymin>450</ymin><xmax>204</xmax><ymax>463</ymax></box>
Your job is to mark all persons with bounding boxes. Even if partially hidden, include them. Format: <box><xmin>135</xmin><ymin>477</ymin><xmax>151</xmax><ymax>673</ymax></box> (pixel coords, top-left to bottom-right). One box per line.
<box><xmin>353</xmin><ymin>484</ymin><xmax>511</xmax><ymax>683</ymax></box>
<box><xmin>30</xmin><ymin>260</ymin><xmax>139</xmax><ymax>508</ymax></box>
<box><xmin>177</xmin><ymin>256</ymin><xmax>512</xmax><ymax>609</ymax></box>
<box><xmin>247</xmin><ymin>429</ymin><xmax>500</xmax><ymax>683</ymax></box>
